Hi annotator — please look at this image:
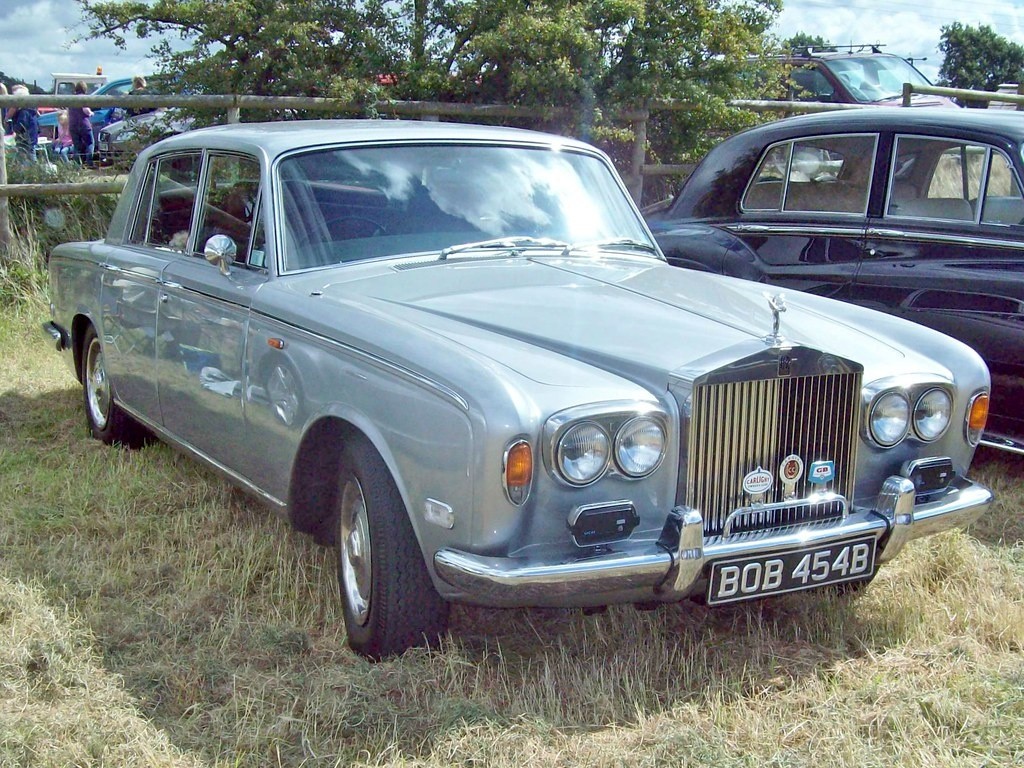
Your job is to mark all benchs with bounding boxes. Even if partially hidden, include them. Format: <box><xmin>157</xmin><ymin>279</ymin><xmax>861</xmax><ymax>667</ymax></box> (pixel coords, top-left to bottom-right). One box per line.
<box><xmin>795</xmin><ymin>180</ymin><xmax>917</xmax><ymax>215</ymax></box>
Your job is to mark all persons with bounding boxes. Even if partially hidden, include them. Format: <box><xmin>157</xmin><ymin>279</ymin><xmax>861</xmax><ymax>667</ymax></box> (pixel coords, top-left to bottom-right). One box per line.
<box><xmin>10</xmin><ymin>84</ymin><xmax>40</xmax><ymax>168</ymax></box>
<box><xmin>123</xmin><ymin>74</ymin><xmax>158</xmax><ymax>116</ymax></box>
<box><xmin>68</xmin><ymin>80</ymin><xmax>97</xmax><ymax>170</ymax></box>
<box><xmin>54</xmin><ymin>112</ymin><xmax>74</xmax><ymax>162</ymax></box>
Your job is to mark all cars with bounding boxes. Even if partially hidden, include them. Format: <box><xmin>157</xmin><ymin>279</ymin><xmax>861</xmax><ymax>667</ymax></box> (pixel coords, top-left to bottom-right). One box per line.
<box><xmin>634</xmin><ymin>105</ymin><xmax>1023</xmax><ymax>457</ymax></box>
<box><xmin>96</xmin><ymin>80</ymin><xmax>306</xmax><ymax>169</ymax></box>
<box><xmin>31</xmin><ymin>72</ymin><xmax>207</xmax><ymax>156</ymax></box>
<box><xmin>42</xmin><ymin>122</ymin><xmax>991</xmax><ymax>664</ymax></box>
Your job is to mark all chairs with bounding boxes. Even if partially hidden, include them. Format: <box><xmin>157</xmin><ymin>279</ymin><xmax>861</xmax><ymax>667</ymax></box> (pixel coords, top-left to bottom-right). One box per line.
<box><xmin>903</xmin><ymin>196</ymin><xmax>975</xmax><ymax>222</ymax></box>
<box><xmin>970</xmin><ymin>196</ymin><xmax>1024</xmax><ymax>224</ymax></box>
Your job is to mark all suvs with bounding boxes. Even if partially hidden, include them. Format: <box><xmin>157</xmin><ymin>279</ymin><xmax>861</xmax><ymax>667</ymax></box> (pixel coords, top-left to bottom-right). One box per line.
<box><xmin>708</xmin><ymin>42</ymin><xmax>963</xmax><ymax>113</ymax></box>
